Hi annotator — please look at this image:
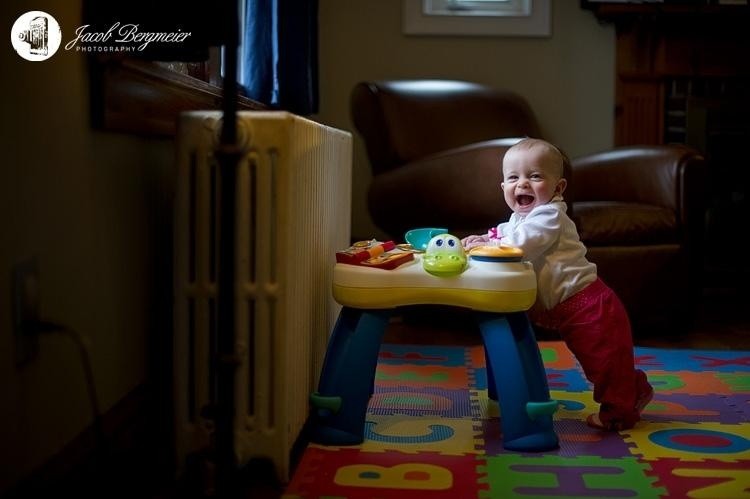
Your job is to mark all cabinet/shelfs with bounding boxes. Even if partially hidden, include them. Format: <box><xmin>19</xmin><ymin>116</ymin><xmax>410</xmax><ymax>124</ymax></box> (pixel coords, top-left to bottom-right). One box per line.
<box><xmin>613</xmin><ymin>19</ymin><xmax>750</xmax><ymax>327</ymax></box>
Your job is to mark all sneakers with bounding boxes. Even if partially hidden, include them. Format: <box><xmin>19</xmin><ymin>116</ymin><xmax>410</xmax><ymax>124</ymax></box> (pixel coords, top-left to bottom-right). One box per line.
<box><xmin>588</xmin><ymin>385</ymin><xmax>656</xmax><ymax>430</ymax></box>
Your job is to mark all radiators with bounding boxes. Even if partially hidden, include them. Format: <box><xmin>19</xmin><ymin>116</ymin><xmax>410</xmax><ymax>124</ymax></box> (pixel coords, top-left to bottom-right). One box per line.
<box><xmin>170</xmin><ymin>109</ymin><xmax>353</xmax><ymax>485</ymax></box>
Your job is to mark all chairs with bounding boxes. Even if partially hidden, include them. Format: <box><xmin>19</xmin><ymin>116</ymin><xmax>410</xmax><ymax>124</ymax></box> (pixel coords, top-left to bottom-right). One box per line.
<box><xmin>351</xmin><ymin>77</ymin><xmax>709</xmax><ymax>337</ymax></box>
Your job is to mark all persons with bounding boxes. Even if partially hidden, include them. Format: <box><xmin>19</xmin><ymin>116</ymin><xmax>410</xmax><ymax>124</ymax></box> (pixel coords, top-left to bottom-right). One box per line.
<box><xmin>459</xmin><ymin>135</ymin><xmax>655</xmax><ymax>431</ymax></box>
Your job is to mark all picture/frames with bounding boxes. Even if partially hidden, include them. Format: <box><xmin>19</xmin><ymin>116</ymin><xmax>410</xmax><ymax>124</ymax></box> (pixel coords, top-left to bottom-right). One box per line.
<box><xmin>401</xmin><ymin>1</ymin><xmax>553</xmax><ymax>38</ymax></box>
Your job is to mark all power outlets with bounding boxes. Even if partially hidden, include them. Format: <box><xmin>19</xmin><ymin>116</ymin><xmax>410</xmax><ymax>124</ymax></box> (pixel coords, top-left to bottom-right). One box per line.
<box><xmin>10</xmin><ymin>256</ymin><xmax>39</xmax><ymax>370</ymax></box>
